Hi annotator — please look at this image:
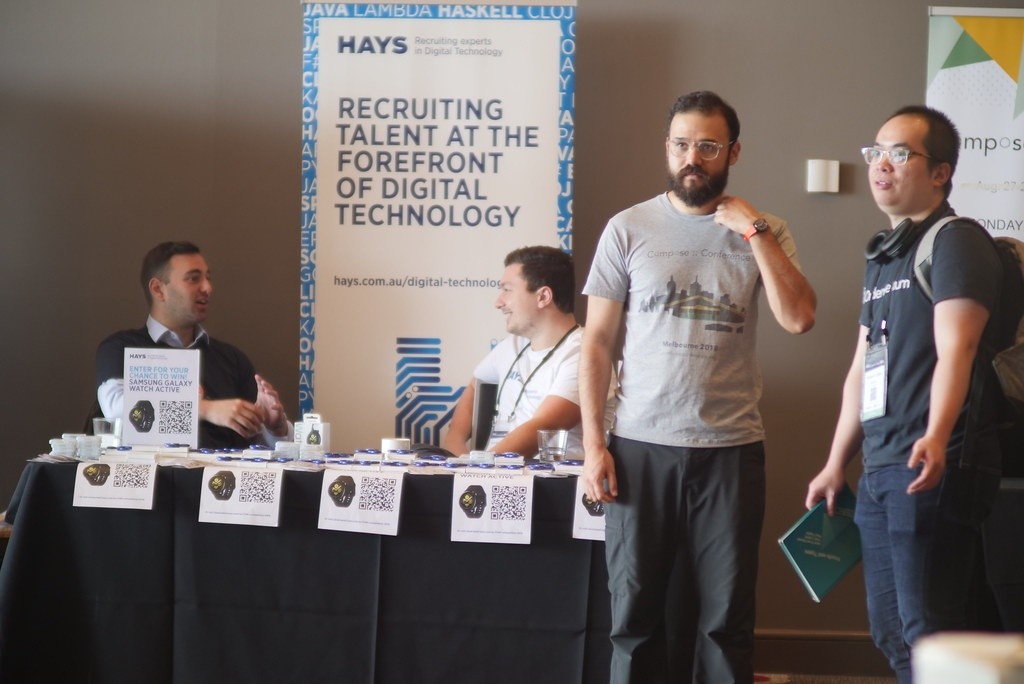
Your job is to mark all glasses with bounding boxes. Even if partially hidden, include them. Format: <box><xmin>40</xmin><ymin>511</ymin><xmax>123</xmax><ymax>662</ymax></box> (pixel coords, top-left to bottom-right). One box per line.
<box><xmin>666</xmin><ymin>139</ymin><xmax>735</xmax><ymax>160</ymax></box>
<box><xmin>860</xmin><ymin>147</ymin><xmax>936</xmax><ymax>166</ymax></box>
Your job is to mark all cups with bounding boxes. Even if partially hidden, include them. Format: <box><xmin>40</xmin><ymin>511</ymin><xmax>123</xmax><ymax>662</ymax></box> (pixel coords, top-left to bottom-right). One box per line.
<box><xmin>381</xmin><ymin>438</ymin><xmax>410</xmax><ymax>453</ymax></box>
<box><xmin>537</xmin><ymin>429</ymin><xmax>568</xmax><ymax>465</ymax></box>
<box><xmin>92</xmin><ymin>418</ymin><xmax>122</xmax><ymax>456</ymax></box>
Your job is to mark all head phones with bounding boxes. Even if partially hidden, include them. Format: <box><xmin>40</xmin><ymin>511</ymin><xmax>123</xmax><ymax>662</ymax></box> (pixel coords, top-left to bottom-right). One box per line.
<box><xmin>865</xmin><ymin>200</ymin><xmax>949</xmax><ymax>264</ymax></box>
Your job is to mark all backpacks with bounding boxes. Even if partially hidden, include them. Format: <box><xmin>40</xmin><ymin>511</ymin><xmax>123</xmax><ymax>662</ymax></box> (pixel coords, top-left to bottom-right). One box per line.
<box><xmin>914</xmin><ymin>215</ymin><xmax>1024</xmax><ymax>406</ymax></box>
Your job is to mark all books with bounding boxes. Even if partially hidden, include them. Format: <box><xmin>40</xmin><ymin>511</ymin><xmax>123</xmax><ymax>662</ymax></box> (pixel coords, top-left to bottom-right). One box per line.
<box><xmin>778</xmin><ymin>480</ymin><xmax>863</xmax><ymax>603</ymax></box>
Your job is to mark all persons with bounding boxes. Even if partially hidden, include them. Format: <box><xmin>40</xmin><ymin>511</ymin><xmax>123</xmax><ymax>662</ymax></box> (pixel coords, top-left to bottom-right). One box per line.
<box><xmin>580</xmin><ymin>89</ymin><xmax>817</xmax><ymax>684</ymax></box>
<box><xmin>805</xmin><ymin>105</ymin><xmax>1006</xmax><ymax>684</ymax></box>
<box><xmin>94</xmin><ymin>241</ymin><xmax>295</xmax><ymax>447</ymax></box>
<box><xmin>445</xmin><ymin>246</ymin><xmax>618</xmax><ymax>462</ymax></box>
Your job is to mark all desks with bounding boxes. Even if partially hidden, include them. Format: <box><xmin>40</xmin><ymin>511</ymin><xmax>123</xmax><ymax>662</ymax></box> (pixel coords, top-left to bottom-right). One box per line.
<box><xmin>0</xmin><ymin>452</ymin><xmax>611</xmax><ymax>684</ymax></box>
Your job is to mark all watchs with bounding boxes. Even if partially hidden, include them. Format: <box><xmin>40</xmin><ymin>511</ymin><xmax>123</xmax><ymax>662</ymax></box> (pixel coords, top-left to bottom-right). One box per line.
<box><xmin>744</xmin><ymin>218</ymin><xmax>771</xmax><ymax>240</ymax></box>
